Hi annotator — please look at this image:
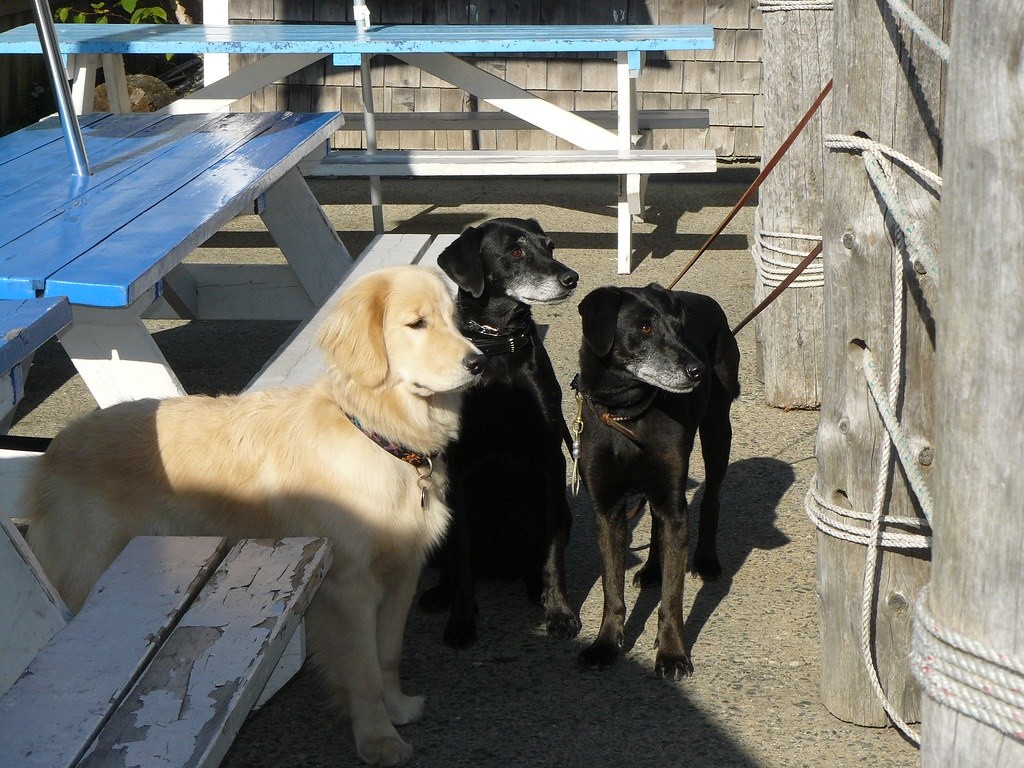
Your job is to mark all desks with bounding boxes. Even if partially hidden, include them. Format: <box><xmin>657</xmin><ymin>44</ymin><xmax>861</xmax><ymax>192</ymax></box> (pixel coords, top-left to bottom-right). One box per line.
<box><xmin>0</xmin><ymin>20</ymin><xmax>714</xmax><ymax>276</ymax></box>
<box><xmin>0</xmin><ymin>109</ymin><xmax>359</xmax><ymax>413</ymax></box>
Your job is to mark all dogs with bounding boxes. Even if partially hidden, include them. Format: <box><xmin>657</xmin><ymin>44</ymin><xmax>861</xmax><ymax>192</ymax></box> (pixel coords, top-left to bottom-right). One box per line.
<box><xmin>16</xmin><ymin>215</ymin><xmax>741</xmax><ymax>768</ymax></box>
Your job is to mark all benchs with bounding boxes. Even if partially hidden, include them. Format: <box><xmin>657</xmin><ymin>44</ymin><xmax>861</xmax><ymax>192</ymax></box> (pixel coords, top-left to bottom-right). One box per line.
<box><xmin>312</xmin><ymin>107</ymin><xmax>718</xmax><ymax>225</ymax></box>
<box><xmin>0</xmin><ymin>536</ymin><xmax>336</xmax><ymax>768</ymax></box>
